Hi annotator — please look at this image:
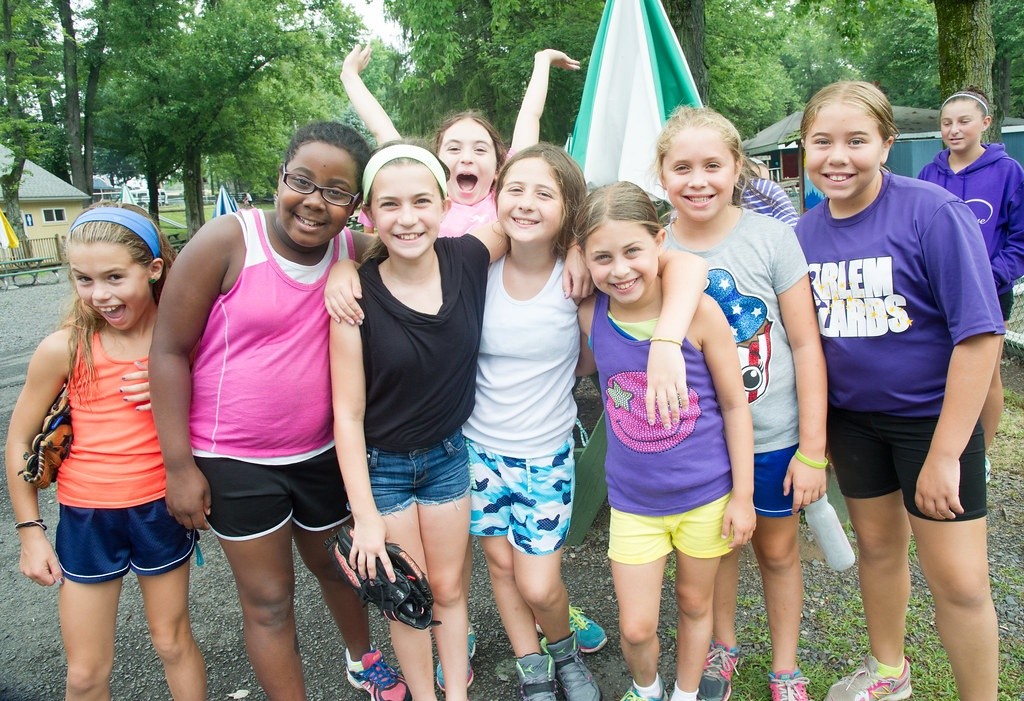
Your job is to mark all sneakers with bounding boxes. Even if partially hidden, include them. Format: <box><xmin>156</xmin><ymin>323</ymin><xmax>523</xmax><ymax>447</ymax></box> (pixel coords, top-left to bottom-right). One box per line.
<box><xmin>513</xmin><ymin>647</ymin><xmax>560</xmax><ymax>701</ymax></box>
<box><xmin>539</xmin><ymin>628</ymin><xmax>601</xmax><ymax>701</ymax></box>
<box><xmin>535</xmin><ymin>603</ymin><xmax>608</xmax><ymax>653</ymax></box>
<box><xmin>825</xmin><ymin>653</ymin><xmax>914</xmax><ymax>701</ymax></box>
<box><xmin>345</xmin><ymin>644</ymin><xmax>413</xmax><ymax>701</ymax></box>
<box><xmin>768</xmin><ymin>668</ymin><xmax>811</xmax><ymax>701</ymax></box>
<box><xmin>693</xmin><ymin>641</ymin><xmax>740</xmax><ymax>701</ymax></box>
<box><xmin>618</xmin><ymin>674</ymin><xmax>670</xmax><ymax>701</ymax></box>
<box><xmin>436</xmin><ymin>620</ymin><xmax>476</xmax><ymax>692</ymax></box>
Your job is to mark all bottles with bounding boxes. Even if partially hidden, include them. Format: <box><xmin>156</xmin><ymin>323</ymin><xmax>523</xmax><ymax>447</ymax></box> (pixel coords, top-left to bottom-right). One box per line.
<box><xmin>803</xmin><ymin>493</ymin><xmax>855</xmax><ymax>570</ymax></box>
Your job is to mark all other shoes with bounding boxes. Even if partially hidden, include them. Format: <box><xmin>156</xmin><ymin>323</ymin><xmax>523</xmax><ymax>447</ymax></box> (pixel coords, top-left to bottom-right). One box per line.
<box><xmin>985</xmin><ymin>456</ymin><xmax>992</xmax><ymax>483</ymax></box>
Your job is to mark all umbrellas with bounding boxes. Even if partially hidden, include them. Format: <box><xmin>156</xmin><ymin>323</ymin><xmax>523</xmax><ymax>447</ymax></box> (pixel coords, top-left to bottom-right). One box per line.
<box><xmin>0</xmin><ymin>207</ymin><xmax>20</xmax><ymax>290</ymax></box>
<box><xmin>212</xmin><ymin>184</ymin><xmax>239</xmax><ymax>218</ymax></box>
<box><xmin>567</xmin><ymin>0</ymin><xmax>706</xmax><ymax>207</ymax></box>
<box><xmin>115</xmin><ymin>185</ymin><xmax>138</xmax><ymax>205</ymax></box>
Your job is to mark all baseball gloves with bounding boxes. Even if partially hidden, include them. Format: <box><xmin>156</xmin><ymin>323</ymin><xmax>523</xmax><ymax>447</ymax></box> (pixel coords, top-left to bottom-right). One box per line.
<box><xmin>322</xmin><ymin>525</ymin><xmax>435</xmax><ymax>630</ymax></box>
<box><xmin>21</xmin><ymin>396</ymin><xmax>73</xmax><ymax>489</ymax></box>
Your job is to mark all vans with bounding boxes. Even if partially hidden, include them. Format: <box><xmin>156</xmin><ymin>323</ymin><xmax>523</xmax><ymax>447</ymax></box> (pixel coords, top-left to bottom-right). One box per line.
<box><xmin>131</xmin><ymin>188</ymin><xmax>166</xmax><ymax>208</ymax></box>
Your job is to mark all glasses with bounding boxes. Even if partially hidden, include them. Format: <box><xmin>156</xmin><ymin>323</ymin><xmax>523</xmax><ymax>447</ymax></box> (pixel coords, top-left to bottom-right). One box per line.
<box><xmin>281</xmin><ymin>161</ymin><xmax>361</xmax><ymax>206</ymax></box>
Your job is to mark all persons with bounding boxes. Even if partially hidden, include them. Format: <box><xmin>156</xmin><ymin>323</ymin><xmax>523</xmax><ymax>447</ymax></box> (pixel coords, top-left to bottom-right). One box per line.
<box><xmin>147</xmin><ymin>44</ymin><xmax>828</xmax><ymax>700</ymax></box>
<box><xmin>4</xmin><ymin>204</ymin><xmax>208</xmax><ymax>701</ymax></box>
<box><xmin>273</xmin><ymin>191</ymin><xmax>278</xmax><ymax>208</ymax></box>
<box><xmin>243</xmin><ymin>191</ymin><xmax>252</xmax><ymax>206</ymax></box>
<box><xmin>792</xmin><ymin>81</ymin><xmax>1024</xmax><ymax>700</ymax></box>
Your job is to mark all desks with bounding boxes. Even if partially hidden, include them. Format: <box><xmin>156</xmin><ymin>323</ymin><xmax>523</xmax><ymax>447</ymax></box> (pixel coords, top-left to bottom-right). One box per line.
<box><xmin>167</xmin><ymin>233</ymin><xmax>184</xmax><ymax>251</ymax></box>
<box><xmin>0</xmin><ymin>255</ymin><xmax>54</xmax><ymax>290</ymax></box>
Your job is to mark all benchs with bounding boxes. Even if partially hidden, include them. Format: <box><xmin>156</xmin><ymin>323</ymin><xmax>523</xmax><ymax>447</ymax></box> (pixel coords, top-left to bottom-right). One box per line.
<box><xmin>170</xmin><ymin>238</ymin><xmax>187</xmax><ymax>248</ymax></box>
<box><xmin>1</xmin><ymin>261</ymin><xmax>67</xmax><ymax>279</ymax></box>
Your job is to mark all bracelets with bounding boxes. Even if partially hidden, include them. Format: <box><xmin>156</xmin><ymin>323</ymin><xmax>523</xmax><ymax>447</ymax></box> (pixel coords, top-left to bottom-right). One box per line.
<box><xmin>649</xmin><ymin>337</ymin><xmax>683</xmax><ymax>348</ymax></box>
<box><xmin>14</xmin><ymin>518</ymin><xmax>47</xmax><ymax>531</ymax></box>
<box><xmin>796</xmin><ymin>448</ymin><xmax>827</xmax><ymax>470</ymax></box>
